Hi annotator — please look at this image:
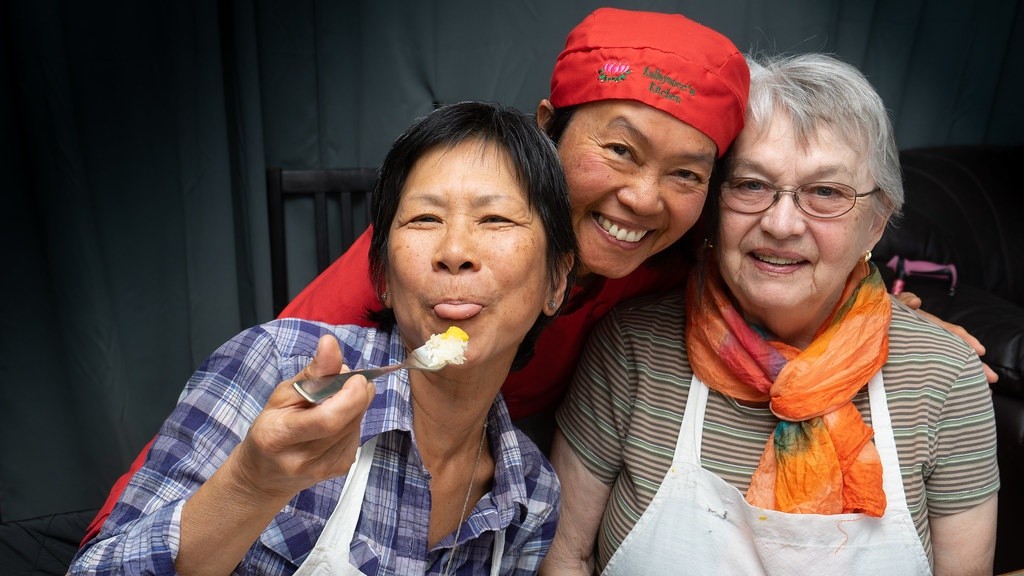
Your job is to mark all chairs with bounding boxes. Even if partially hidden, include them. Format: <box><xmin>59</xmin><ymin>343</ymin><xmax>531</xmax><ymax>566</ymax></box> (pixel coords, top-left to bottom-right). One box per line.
<box><xmin>266</xmin><ymin>164</ymin><xmax>381</xmax><ymax>320</ymax></box>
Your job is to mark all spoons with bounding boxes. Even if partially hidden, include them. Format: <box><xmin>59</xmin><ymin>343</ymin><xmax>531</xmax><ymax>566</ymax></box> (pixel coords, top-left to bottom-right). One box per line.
<box><xmin>292</xmin><ymin>339</ymin><xmax>450</xmax><ymax>404</ymax></box>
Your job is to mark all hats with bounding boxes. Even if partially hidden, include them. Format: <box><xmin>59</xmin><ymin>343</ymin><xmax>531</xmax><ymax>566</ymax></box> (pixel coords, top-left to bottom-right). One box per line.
<box><xmin>550</xmin><ymin>7</ymin><xmax>750</xmax><ymax>159</ymax></box>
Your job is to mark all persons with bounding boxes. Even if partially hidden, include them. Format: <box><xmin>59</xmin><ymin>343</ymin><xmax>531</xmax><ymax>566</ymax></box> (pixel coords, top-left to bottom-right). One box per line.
<box><xmin>67</xmin><ymin>99</ymin><xmax>581</xmax><ymax>576</ymax></box>
<box><xmin>68</xmin><ymin>8</ymin><xmax>1001</xmax><ymax>562</ymax></box>
<box><xmin>537</xmin><ymin>51</ymin><xmax>1002</xmax><ymax>576</ymax></box>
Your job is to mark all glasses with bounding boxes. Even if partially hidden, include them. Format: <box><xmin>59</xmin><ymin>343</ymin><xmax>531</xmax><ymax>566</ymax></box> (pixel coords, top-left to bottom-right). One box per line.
<box><xmin>718</xmin><ymin>177</ymin><xmax>879</xmax><ymax>218</ymax></box>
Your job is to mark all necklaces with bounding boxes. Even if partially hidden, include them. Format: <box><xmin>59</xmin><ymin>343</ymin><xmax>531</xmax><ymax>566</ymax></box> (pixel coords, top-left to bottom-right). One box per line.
<box><xmin>444</xmin><ymin>416</ymin><xmax>489</xmax><ymax>576</ymax></box>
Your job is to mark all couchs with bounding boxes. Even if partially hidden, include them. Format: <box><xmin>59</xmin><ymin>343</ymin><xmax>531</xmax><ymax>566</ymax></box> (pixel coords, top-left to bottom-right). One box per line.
<box><xmin>870</xmin><ymin>143</ymin><xmax>1024</xmax><ymax>576</ymax></box>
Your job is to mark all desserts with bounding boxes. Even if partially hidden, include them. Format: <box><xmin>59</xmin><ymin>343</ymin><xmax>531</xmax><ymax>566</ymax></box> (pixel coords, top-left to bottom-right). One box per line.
<box><xmin>425</xmin><ymin>326</ymin><xmax>470</xmax><ymax>367</ymax></box>
